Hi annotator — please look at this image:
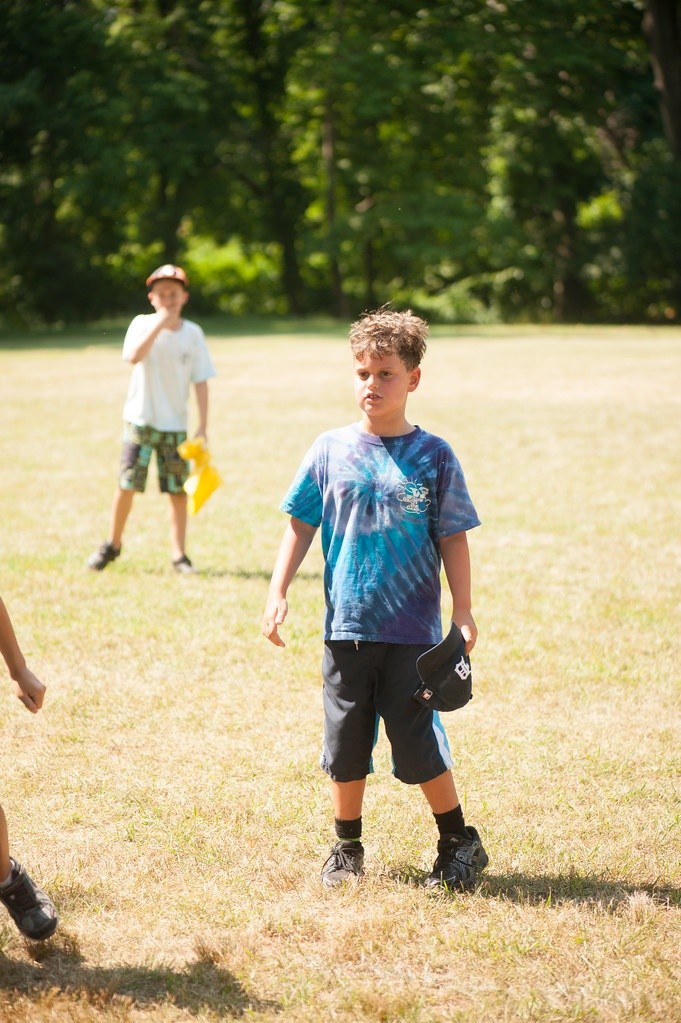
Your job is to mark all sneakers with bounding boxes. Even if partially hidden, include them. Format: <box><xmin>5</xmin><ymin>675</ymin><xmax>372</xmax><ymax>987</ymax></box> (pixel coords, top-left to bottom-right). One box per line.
<box><xmin>0</xmin><ymin>856</ymin><xmax>59</xmax><ymax>940</ymax></box>
<box><xmin>429</xmin><ymin>825</ymin><xmax>488</xmax><ymax>891</ymax></box>
<box><xmin>321</xmin><ymin>839</ymin><xmax>364</xmax><ymax>886</ymax></box>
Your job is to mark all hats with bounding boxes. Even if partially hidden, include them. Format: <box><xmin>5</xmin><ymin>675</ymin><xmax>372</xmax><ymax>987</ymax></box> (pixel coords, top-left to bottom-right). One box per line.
<box><xmin>146</xmin><ymin>264</ymin><xmax>189</xmax><ymax>288</ymax></box>
<box><xmin>412</xmin><ymin>621</ymin><xmax>473</xmax><ymax>713</ymax></box>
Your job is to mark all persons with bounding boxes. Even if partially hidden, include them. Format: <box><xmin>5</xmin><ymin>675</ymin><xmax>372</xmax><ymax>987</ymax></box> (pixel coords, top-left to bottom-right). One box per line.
<box><xmin>89</xmin><ymin>265</ymin><xmax>217</xmax><ymax>574</ymax></box>
<box><xmin>0</xmin><ymin>597</ymin><xmax>62</xmax><ymax>938</ymax></box>
<box><xmin>263</xmin><ymin>308</ymin><xmax>490</xmax><ymax>895</ymax></box>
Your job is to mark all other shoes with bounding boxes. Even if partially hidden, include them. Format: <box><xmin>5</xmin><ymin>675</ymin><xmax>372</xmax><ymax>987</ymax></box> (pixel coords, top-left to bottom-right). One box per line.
<box><xmin>172</xmin><ymin>554</ymin><xmax>193</xmax><ymax>572</ymax></box>
<box><xmin>87</xmin><ymin>541</ymin><xmax>122</xmax><ymax>571</ymax></box>
